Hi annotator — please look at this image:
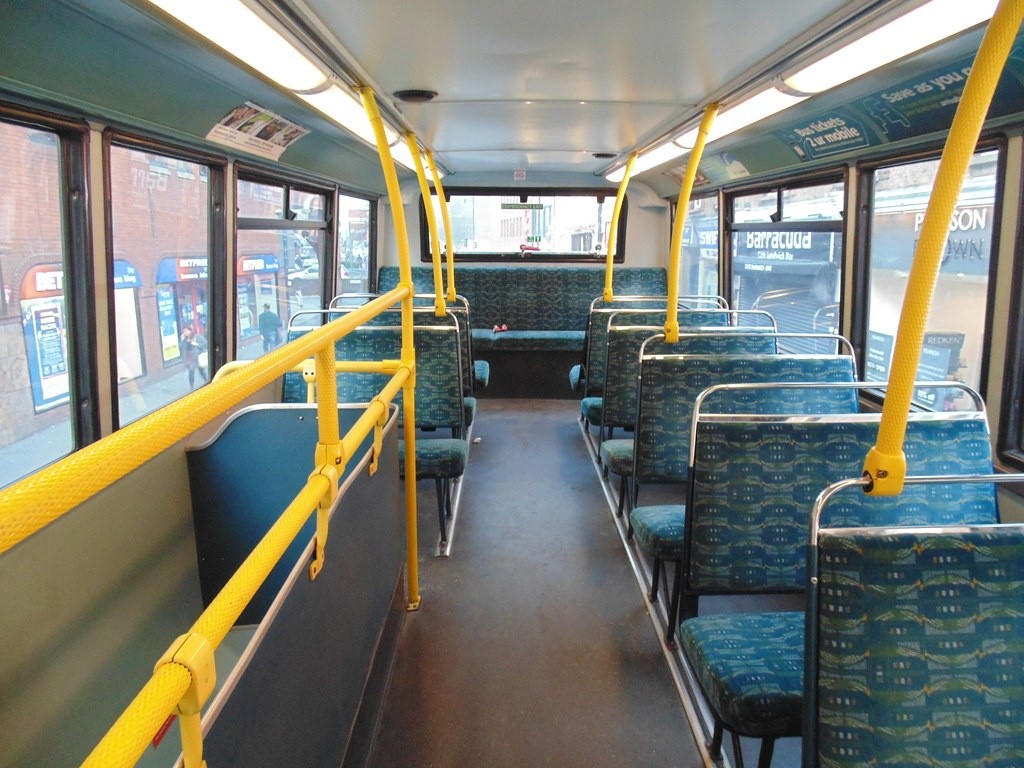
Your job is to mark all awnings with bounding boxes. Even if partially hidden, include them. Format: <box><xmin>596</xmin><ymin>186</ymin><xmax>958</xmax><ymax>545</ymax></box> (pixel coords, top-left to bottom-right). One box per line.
<box><xmin>732</xmin><ymin>255</ymin><xmax>836</xmax><ymax>281</ymax></box>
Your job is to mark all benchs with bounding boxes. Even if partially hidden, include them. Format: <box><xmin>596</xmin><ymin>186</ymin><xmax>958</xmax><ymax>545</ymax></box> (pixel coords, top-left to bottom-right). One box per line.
<box><xmin>282</xmin><ymin>293</ymin><xmax>490</xmax><ymax>544</ymax></box>
<box><xmin>378</xmin><ymin>266</ymin><xmax>668</xmax><ymax>351</ymax></box>
<box><xmin>570</xmin><ymin>296</ymin><xmax>1024</xmax><ymax>768</ymax></box>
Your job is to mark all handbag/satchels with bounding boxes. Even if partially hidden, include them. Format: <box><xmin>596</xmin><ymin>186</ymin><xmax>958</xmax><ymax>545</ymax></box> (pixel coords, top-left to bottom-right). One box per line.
<box><xmin>198</xmin><ymin>351</ymin><xmax>208</xmax><ymax>368</ymax></box>
<box><xmin>276</xmin><ymin>327</ymin><xmax>282</xmax><ymax>346</ymax></box>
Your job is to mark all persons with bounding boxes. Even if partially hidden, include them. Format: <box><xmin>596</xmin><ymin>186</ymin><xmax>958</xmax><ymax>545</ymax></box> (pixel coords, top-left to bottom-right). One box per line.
<box><xmin>181</xmin><ymin>327</ymin><xmax>209</xmax><ymax>390</ymax></box>
<box><xmin>356</xmin><ymin>254</ymin><xmax>363</xmax><ymax>269</ymax></box>
<box><xmin>259</xmin><ymin>304</ymin><xmax>281</xmax><ymax>353</ymax></box>
